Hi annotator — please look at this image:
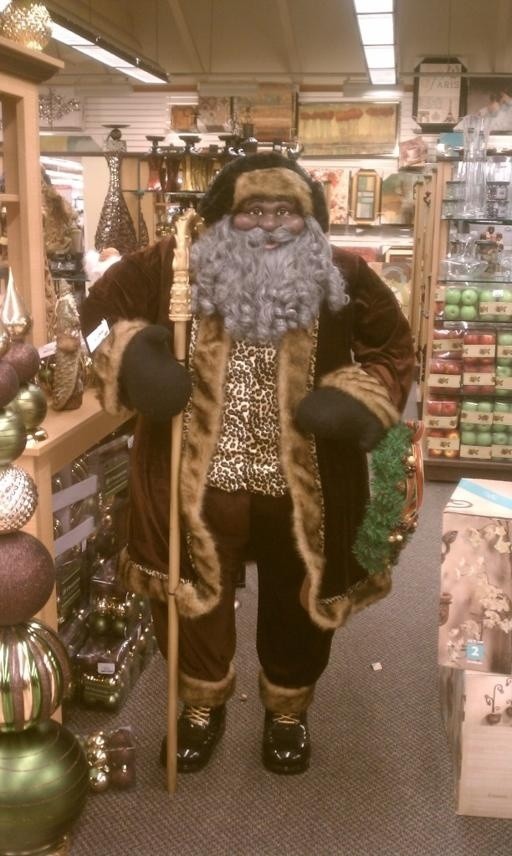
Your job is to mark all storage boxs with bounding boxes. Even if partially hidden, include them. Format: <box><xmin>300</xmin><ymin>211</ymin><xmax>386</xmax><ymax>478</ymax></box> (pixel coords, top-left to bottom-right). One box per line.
<box><xmin>437</xmin><ymin>475</ymin><xmax>512</xmax><ymax>821</ymax></box>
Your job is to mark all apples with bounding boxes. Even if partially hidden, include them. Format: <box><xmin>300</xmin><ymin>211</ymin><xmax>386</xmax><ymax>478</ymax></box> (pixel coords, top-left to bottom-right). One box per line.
<box><xmin>425</xmin><ymin>287</ymin><xmax>512</xmax><ymax>461</ymax></box>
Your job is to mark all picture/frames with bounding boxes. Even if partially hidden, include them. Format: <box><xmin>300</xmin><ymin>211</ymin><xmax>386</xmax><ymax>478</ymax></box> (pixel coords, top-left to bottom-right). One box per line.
<box><xmin>348</xmin><ymin>166</ymin><xmax>384</xmax><ymax>228</ymax></box>
<box><xmin>411</xmin><ymin>54</ymin><xmax>471</xmax><ymax>135</ymax></box>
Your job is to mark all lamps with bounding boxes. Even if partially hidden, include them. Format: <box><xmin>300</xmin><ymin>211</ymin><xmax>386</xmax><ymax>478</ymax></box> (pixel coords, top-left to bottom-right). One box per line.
<box><xmin>43</xmin><ymin>12</ymin><xmax>169</xmax><ymax>90</ymax></box>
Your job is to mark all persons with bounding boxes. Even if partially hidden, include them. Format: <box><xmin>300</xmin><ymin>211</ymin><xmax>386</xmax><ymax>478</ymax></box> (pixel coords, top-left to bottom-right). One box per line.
<box><xmin>75</xmin><ymin>150</ymin><xmax>421</xmax><ymax>777</ymax></box>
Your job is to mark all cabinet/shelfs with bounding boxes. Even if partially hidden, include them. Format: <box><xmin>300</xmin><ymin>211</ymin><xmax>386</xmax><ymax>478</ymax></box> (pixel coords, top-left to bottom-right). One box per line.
<box><xmin>410</xmin><ymin>148</ymin><xmax>512</xmax><ymax>484</ymax></box>
<box><xmin>0</xmin><ymin>31</ymin><xmax>133</xmax><ymax>727</ymax></box>
